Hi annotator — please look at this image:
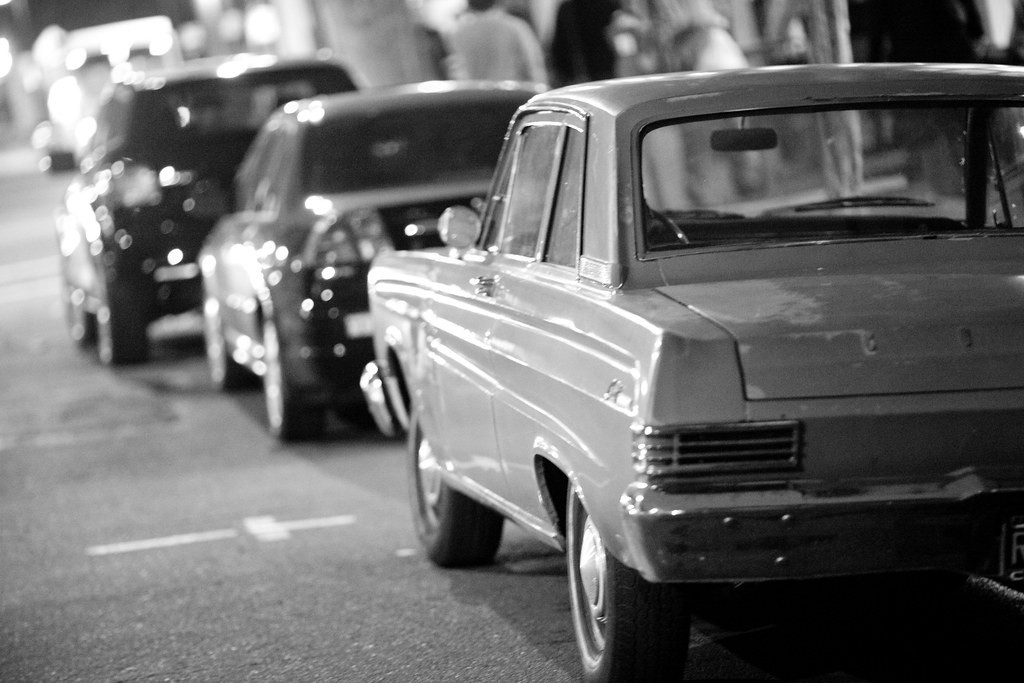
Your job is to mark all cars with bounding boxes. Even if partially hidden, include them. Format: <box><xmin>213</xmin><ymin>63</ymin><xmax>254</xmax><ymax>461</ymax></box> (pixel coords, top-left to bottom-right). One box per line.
<box><xmin>357</xmin><ymin>61</ymin><xmax>1024</xmax><ymax>683</ymax></box>
<box><xmin>39</xmin><ymin>58</ymin><xmax>356</xmax><ymax>365</ymax></box>
<box><xmin>196</xmin><ymin>80</ymin><xmax>554</xmax><ymax>437</ymax></box>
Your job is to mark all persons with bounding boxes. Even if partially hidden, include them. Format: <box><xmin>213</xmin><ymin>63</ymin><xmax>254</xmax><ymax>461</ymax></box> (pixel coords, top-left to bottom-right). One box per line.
<box><xmin>848</xmin><ymin>0</ymin><xmax>984</xmax><ymax>179</ymax></box>
<box><xmin>453</xmin><ymin>0</ymin><xmax>622</xmax><ymax>86</ymax></box>
